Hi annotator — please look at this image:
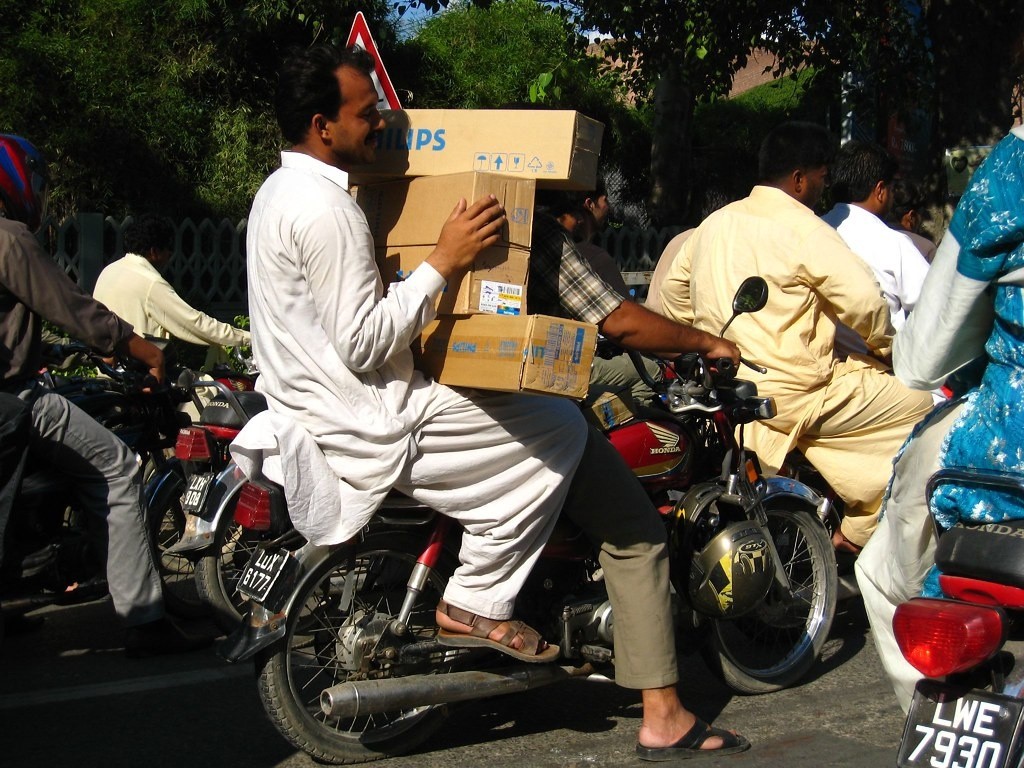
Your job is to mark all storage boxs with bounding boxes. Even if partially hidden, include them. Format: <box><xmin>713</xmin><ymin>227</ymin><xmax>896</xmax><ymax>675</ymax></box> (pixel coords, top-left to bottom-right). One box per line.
<box><xmin>343</xmin><ymin>105</ymin><xmax>608</xmax><ymax>191</ymax></box>
<box><xmin>355</xmin><ymin>172</ymin><xmax>568</xmax><ymax>258</ymax></box>
<box><xmin>579</xmin><ymin>382</ymin><xmax>639</xmax><ymax>432</ymax></box>
<box><xmin>368</xmin><ymin>244</ymin><xmax>560</xmax><ymax>317</ymax></box>
<box><xmin>408</xmin><ymin>314</ymin><xmax>599</xmax><ymax>401</ymax></box>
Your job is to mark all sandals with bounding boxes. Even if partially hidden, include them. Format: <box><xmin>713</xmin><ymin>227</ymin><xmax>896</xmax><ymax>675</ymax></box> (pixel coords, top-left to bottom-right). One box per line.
<box><xmin>435</xmin><ymin>599</ymin><xmax>560</xmax><ymax>662</ymax></box>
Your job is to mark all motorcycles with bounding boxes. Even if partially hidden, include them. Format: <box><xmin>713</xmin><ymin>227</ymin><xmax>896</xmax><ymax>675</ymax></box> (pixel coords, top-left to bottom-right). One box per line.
<box><xmin>1</xmin><ymin>274</ymin><xmax>848</xmax><ymax>764</ymax></box>
<box><xmin>891</xmin><ymin>356</ymin><xmax>1024</xmax><ymax>767</ymax></box>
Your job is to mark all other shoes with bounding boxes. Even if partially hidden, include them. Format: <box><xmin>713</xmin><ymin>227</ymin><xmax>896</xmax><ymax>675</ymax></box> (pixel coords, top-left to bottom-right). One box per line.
<box><xmin>124</xmin><ymin>621</ymin><xmax>213</xmax><ymax>662</ymax></box>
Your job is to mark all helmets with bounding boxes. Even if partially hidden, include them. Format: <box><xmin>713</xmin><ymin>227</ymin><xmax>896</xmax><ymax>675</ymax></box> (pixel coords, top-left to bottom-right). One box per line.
<box><xmin>669</xmin><ymin>480</ymin><xmax>775</xmax><ymax>620</ymax></box>
<box><xmin>1</xmin><ymin>135</ymin><xmax>54</xmax><ymax>236</ymax></box>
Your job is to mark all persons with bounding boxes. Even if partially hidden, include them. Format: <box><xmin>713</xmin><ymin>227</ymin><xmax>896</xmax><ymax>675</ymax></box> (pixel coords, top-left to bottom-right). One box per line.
<box><xmin>551</xmin><ymin>120</ymin><xmax>1024</xmax><ymax>762</ymax></box>
<box><xmin>92</xmin><ymin>219</ymin><xmax>253</xmax><ymax>424</ymax></box>
<box><xmin>0</xmin><ymin>134</ymin><xmax>215</xmax><ymax>660</ymax></box>
<box><xmin>228</xmin><ymin>43</ymin><xmax>561</xmax><ymax>662</ymax></box>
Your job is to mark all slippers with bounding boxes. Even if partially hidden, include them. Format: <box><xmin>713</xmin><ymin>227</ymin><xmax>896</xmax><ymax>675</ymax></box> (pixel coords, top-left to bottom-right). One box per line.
<box><xmin>636</xmin><ymin>713</ymin><xmax>751</xmax><ymax>760</ymax></box>
